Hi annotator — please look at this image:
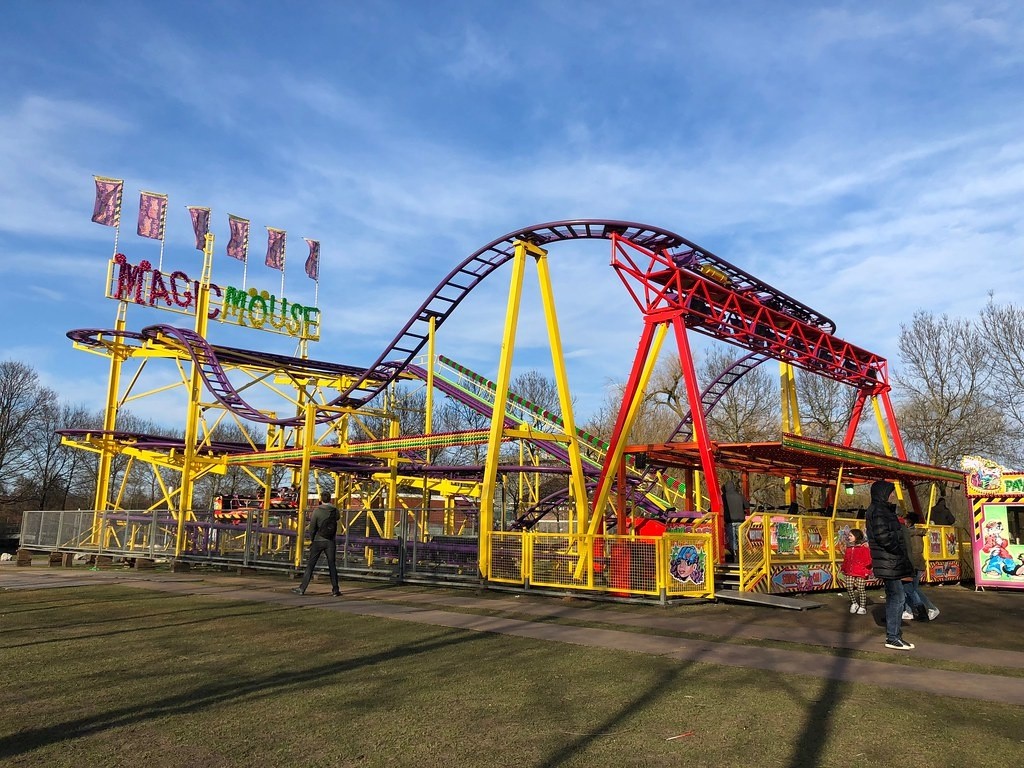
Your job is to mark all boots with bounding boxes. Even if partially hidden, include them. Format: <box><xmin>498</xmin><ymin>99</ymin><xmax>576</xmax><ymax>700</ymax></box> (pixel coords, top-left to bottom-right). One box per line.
<box><xmin>881</xmin><ymin>617</ymin><xmax>887</xmax><ymax>622</ymax></box>
<box><xmin>914</xmin><ymin>603</ymin><xmax>929</xmax><ymax>622</ymax></box>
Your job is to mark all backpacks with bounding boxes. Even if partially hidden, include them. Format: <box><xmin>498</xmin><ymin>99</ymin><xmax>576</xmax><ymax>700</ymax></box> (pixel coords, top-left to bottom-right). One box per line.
<box><xmin>317</xmin><ymin>508</ymin><xmax>337</xmax><ymax>541</ymax></box>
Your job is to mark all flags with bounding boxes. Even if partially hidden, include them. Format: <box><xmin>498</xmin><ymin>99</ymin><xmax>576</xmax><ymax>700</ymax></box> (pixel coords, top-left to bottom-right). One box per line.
<box><xmin>91</xmin><ymin>175</ymin><xmax>321</xmax><ymax>281</ymax></box>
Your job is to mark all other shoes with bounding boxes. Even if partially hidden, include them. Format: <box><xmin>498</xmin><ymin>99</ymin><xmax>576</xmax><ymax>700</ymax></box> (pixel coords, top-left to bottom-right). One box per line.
<box><xmin>333</xmin><ymin>591</ymin><xmax>341</xmax><ymax>597</ymax></box>
<box><xmin>291</xmin><ymin>588</ymin><xmax>304</xmax><ymax>596</ymax></box>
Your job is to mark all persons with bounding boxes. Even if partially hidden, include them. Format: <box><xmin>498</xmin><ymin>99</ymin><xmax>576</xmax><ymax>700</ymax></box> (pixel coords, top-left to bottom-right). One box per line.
<box><xmin>866</xmin><ymin>481</ymin><xmax>915</xmax><ymax>649</ymax></box>
<box><xmin>276</xmin><ymin>486</ymin><xmax>299</xmax><ymax>499</ymax></box>
<box><xmin>708</xmin><ymin>480</ymin><xmax>750</xmax><ymax>563</ymax></box>
<box><xmin>902</xmin><ymin>516</ymin><xmax>940</xmax><ymax>620</ymax></box>
<box><xmin>926</xmin><ymin>498</ymin><xmax>955</xmax><ymax>525</ymax></box>
<box><xmin>291</xmin><ymin>491</ymin><xmax>341</xmax><ymax>597</ymax></box>
<box><xmin>841</xmin><ymin>528</ymin><xmax>873</xmax><ymax>614</ymax></box>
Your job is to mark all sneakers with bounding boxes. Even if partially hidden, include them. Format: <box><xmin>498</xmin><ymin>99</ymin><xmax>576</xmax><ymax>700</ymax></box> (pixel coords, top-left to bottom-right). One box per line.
<box><xmin>899</xmin><ymin>637</ymin><xmax>915</xmax><ymax>648</ymax></box>
<box><xmin>901</xmin><ymin>611</ymin><xmax>914</xmax><ymax>620</ymax></box>
<box><xmin>849</xmin><ymin>603</ymin><xmax>859</xmax><ymax>613</ymax></box>
<box><xmin>927</xmin><ymin>607</ymin><xmax>940</xmax><ymax>620</ymax></box>
<box><xmin>857</xmin><ymin>607</ymin><xmax>866</xmax><ymax>614</ymax></box>
<box><xmin>885</xmin><ymin>639</ymin><xmax>911</xmax><ymax>651</ymax></box>
